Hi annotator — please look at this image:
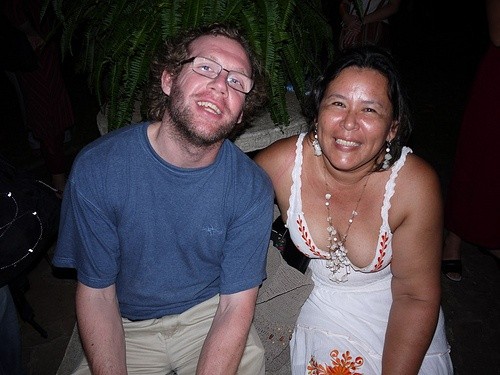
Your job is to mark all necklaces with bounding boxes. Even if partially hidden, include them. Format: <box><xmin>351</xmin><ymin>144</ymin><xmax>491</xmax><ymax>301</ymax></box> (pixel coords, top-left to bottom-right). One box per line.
<box><xmin>322</xmin><ymin>151</ymin><xmax>377</xmax><ymax>283</ymax></box>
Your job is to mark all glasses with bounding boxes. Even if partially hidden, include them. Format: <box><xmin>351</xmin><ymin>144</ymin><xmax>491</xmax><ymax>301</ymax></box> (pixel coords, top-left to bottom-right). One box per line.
<box><xmin>177</xmin><ymin>56</ymin><xmax>255</xmax><ymax>94</ymax></box>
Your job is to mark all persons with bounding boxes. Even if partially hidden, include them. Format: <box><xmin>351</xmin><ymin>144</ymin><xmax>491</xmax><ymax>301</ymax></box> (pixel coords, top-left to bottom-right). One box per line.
<box><xmin>49</xmin><ymin>23</ymin><xmax>275</xmax><ymax>375</ymax></box>
<box><xmin>253</xmin><ymin>49</ymin><xmax>454</xmax><ymax>375</ymax></box>
<box><xmin>337</xmin><ymin>0</ymin><xmax>400</xmax><ymax>52</ymax></box>
<box><xmin>443</xmin><ymin>0</ymin><xmax>500</xmax><ymax>283</ymax></box>
